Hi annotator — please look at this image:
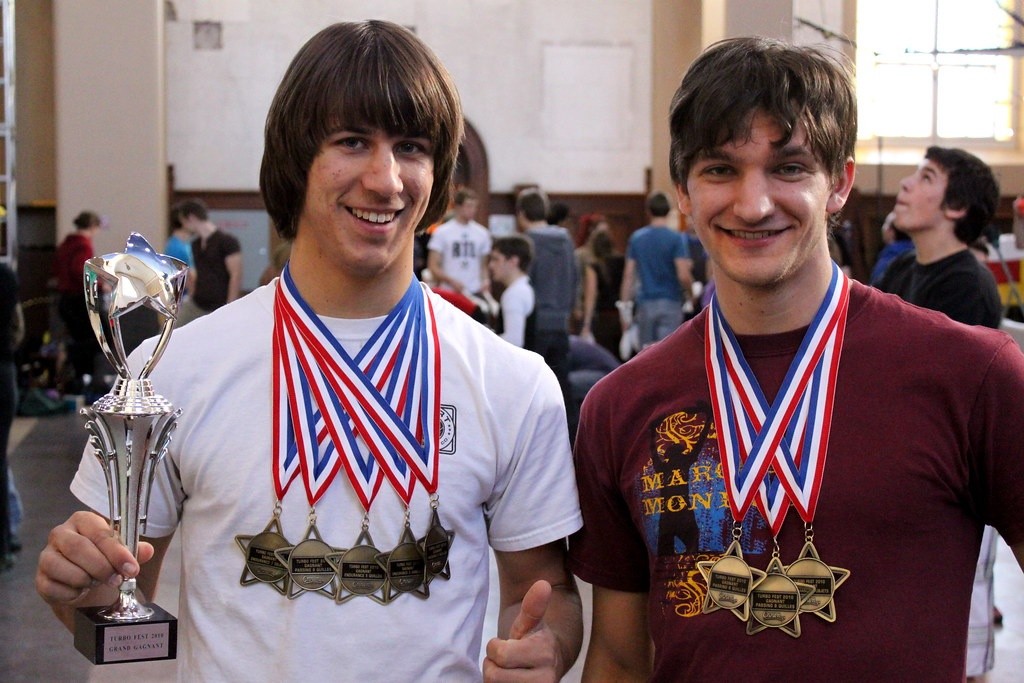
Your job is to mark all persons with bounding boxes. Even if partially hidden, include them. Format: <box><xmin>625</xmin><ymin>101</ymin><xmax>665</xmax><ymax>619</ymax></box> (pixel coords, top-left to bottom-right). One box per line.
<box><xmin>33</xmin><ymin>18</ymin><xmax>584</xmax><ymax>683</ymax></box>
<box><xmin>0</xmin><ymin>262</ymin><xmax>25</xmax><ymax>563</ymax></box>
<box><xmin>569</xmin><ymin>37</ymin><xmax>1024</xmax><ymax>683</ymax></box>
<box><xmin>56</xmin><ymin>199</ymin><xmax>243</xmax><ymax>394</ymax></box>
<box><xmin>427</xmin><ymin>147</ymin><xmax>1003</xmax><ymax>399</ymax></box>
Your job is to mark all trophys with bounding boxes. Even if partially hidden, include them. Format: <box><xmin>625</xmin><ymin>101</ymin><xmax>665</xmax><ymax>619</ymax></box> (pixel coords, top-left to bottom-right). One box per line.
<box><xmin>73</xmin><ymin>231</ymin><xmax>189</xmax><ymax>666</ymax></box>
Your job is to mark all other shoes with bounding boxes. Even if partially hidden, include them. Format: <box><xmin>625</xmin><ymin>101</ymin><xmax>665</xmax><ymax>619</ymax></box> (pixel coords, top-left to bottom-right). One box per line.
<box><xmin>1</xmin><ymin>533</ymin><xmax>22</xmax><ymax>578</ymax></box>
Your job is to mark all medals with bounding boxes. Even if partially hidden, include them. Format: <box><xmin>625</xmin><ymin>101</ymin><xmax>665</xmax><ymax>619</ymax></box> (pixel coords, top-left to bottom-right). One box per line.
<box><xmin>234</xmin><ymin>507</ymin><xmax>455</xmax><ymax>606</ymax></box>
<box><xmin>696</xmin><ymin>541</ymin><xmax>850</xmax><ymax>638</ymax></box>
